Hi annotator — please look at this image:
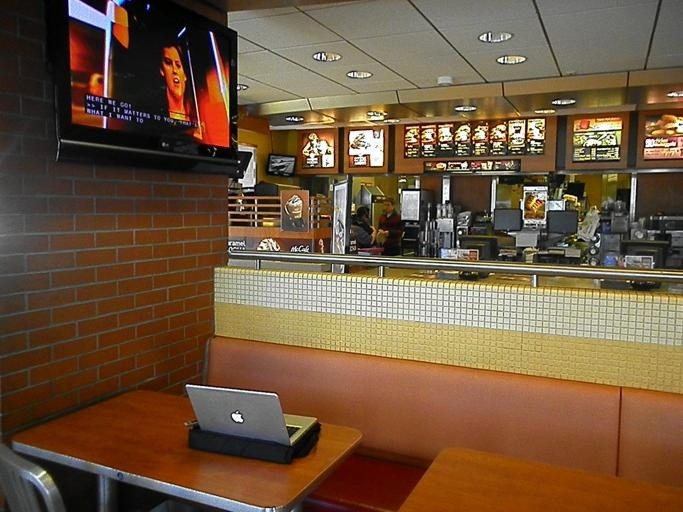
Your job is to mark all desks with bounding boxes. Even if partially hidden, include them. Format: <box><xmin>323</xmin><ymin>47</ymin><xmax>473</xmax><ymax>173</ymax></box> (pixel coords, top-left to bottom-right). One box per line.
<box><xmin>356</xmin><ymin>267</ymin><xmax>602</xmax><ymax>290</ymax></box>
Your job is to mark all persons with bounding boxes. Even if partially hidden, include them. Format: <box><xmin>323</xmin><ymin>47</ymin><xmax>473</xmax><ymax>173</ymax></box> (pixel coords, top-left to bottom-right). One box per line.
<box><xmin>350</xmin><ymin>206</ymin><xmax>374</xmax><ymax>249</ymax></box>
<box><xmin>376</xmin><ymin>197</ymin><xmax>402</xmax><ymax>256</ymax></box>
<box><xmin>149</xmin><ymin>41</ymin><xmax>192</xmax><ymax>122</ymax></box>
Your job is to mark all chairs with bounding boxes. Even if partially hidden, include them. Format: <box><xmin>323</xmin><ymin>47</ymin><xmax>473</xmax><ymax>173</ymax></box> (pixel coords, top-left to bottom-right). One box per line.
<box><xmin>0</xmin><ymin>442</ymin><xmax>66</xmax><ymax>512</ymax></box>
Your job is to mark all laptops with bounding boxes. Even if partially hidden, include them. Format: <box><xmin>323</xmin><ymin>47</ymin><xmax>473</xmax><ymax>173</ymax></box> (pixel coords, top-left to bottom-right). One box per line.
<box><xmin>185</xmin><ymin>384</ymin><xmax>322</xmax><ymax>447</ymax></box>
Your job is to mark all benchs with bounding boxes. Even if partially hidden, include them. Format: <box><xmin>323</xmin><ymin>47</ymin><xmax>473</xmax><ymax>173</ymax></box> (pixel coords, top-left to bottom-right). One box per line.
<box><xmin>618</xmin><ymin>386</ymin><xmax>683</xmax><ymax>486</ymax></box>
<box><xmin>206</xmin><ymin>336</ymin><xmax>621</xmax><ymax>512</ymax></box>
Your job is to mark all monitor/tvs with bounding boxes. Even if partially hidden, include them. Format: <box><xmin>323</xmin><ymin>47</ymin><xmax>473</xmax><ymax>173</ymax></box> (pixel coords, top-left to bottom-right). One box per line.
<box><xmin>265</xmin><ymin>153</ymin><xmax>297</xmax><ymax>177</ymax></box>
<box><xmin>460</xmin><ymin>237</ymin><xmax>497</xmax><ymax>278</ymax></box>
<box><xmin>620</xmin><ymin>239</ymin><xmax>669</xmax><ymax>289</ymax></box>
<box><xmin>547</xmin><ymin>210</ymin><xmax>578</xmax><ymax>235</ymax></box>
<box><xmin>493</xmin><ymin>208</ymin><xmax>523</xmax><ymax>232</ymax></box>
<box><xmin>54</xmin><ymin>0</ymin><xmax>239</xmax><ymax>174</ymax></box>
